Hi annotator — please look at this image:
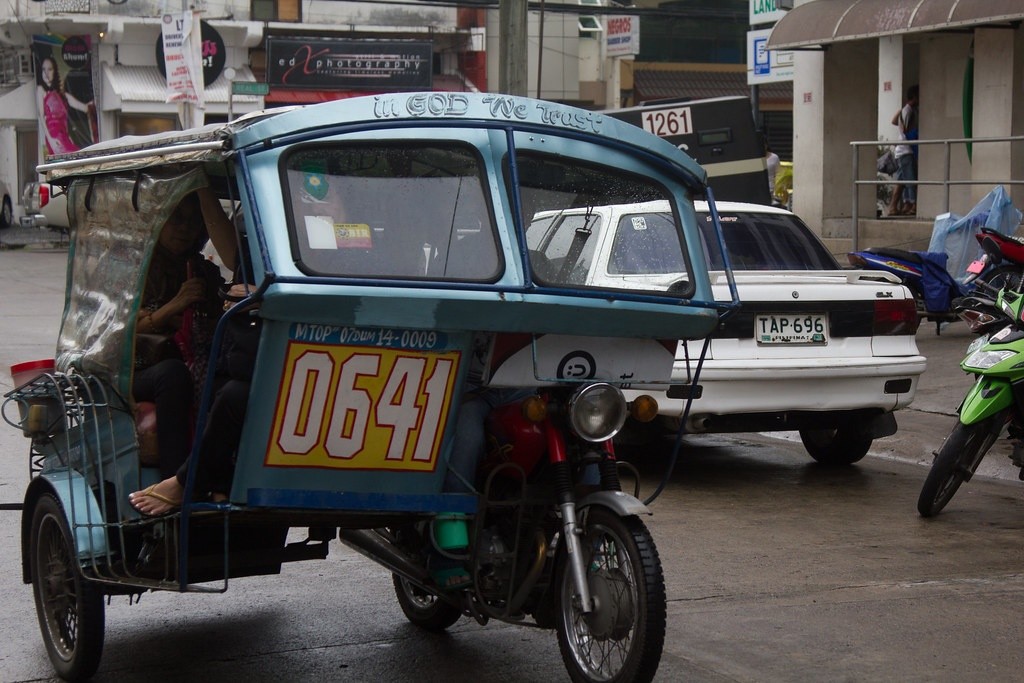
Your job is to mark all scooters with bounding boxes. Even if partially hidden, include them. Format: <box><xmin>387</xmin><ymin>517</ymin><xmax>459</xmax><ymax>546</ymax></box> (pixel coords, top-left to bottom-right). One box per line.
<box><xmin>846</xmin><ymin>186</ymin><xmax>1015</xmax><ymax>334</ymax></box>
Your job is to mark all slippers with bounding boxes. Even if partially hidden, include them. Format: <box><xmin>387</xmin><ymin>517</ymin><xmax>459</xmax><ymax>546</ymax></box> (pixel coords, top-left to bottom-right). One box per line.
<box><xmin>129</xmin><ymin>482</ymin><xmax>203</xmax><ymax>519</ymax></box>
<box><xmin>881</xmin><ymin>204</ymin><xmax>916</xmax><ymax>217</ymax></box>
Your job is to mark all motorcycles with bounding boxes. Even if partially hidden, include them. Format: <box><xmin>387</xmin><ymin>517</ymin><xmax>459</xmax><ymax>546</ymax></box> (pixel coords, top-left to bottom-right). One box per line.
<box><xmin>916</xmin><ymin>237</ymin><xmax>1024</xmax><ymax>517</ymax></box>
<box><xmin>962</xmin><ymin>227</ymin><xmax>1024</xmax><ymax>323</ymax></box>
<box><xmin>1</xmin><ymin>91</ymin><xmax>742</xmax><ymax>683</ymax></box>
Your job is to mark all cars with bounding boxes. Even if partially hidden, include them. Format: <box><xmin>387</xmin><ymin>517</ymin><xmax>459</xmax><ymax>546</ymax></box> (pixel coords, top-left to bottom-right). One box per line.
<box><xmin>525</xmin><ymin>201</ymin><xmax>929</xmax><ymax>465</ymax></box>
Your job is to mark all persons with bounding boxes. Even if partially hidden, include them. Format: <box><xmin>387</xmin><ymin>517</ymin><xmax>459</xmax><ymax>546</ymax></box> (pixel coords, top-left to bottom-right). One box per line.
<box><xmin>886</xmin><ymin>84</ymin><xmax>919</xmax><ymax>215</ymax></box>
<box><xmin>431</xmin><ymin>176</ymin><xmax>559</xmax><ymax>283</ymax></box>
<box><xmin>765</xmin><ymin>136</ymin><xmax>780</xmax><ymax>198</ymax></box>
<box><xmin>35</xmin><ymin>53</ymin><xmax>92</xmax><ymax>157</ymax></box>
<box><xmin>125</xmin><ymin>165</ymin><xmax>262</xmax><ymax>514</ymax></box>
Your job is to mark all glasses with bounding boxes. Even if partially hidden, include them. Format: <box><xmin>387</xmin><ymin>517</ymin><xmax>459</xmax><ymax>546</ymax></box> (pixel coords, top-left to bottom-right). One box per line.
<box><xmin>167</xmin><ymin>208</ymin><xmax>204</xmax><ymax>234</ymax></box>
